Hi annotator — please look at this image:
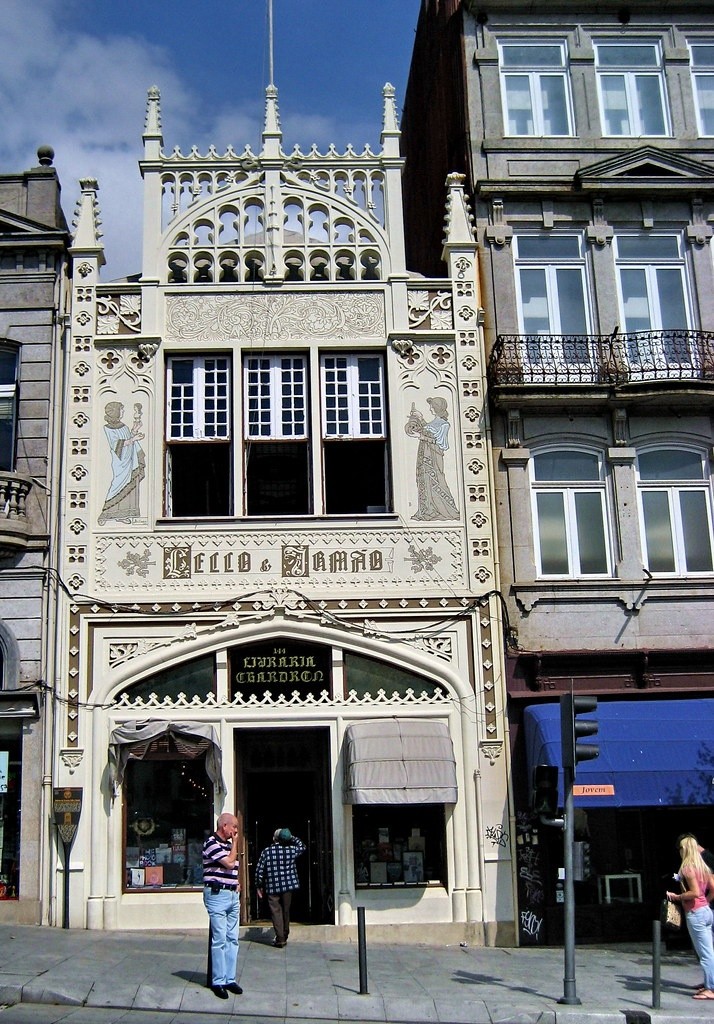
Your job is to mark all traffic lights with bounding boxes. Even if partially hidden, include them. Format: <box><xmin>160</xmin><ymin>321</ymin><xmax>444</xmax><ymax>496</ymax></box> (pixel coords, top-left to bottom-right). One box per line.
<box><xmin>573</xmin><ymin>693</ymin><xmax>599</xmax><ymax>766</ymax></box>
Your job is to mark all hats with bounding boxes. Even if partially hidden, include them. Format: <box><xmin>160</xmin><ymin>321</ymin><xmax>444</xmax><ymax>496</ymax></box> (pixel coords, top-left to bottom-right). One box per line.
<box><xmin>279</xmin><ymin>828</ymin><xmax>291</xmax><ymax>843</ymax></box>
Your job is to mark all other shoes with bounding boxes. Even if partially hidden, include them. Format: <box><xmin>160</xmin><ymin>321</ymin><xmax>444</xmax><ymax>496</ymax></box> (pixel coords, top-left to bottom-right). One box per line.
<box><xmin>275</xmin><ymin>941</ymin><xmax>288</xmax><ymax>948</ymax></box>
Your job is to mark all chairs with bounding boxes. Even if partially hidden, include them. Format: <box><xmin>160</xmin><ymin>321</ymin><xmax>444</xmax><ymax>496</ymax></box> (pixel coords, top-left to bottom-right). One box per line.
<box><xmin>598</xmin><ymin>342</ymin><xmax>629</xmax><ymax>383</ymax></box>
<box><xmin>495</xmin><ymin>347</ymin><xmax>524</xmax><ymax>382</ymax></box>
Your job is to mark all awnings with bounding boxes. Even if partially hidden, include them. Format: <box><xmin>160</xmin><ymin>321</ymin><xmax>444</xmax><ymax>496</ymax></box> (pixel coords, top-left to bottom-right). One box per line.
<box><xmin>108</xmin><ymin>717</ymin><xmax>227</xmax><ymax>807</ymax></box>
<box><xmin>344</xmin><ymin>721</ymin><xmax>459</xmax><ymax>804</ymax></box>
<box><xmin>523</xmin><ymin>701</ymin><xmax>714</xmax><ymax>809</ymax></box>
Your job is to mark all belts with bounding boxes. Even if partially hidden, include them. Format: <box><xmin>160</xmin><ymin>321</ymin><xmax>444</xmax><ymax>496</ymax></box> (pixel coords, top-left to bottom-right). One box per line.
<box><xmin>205</xmin><ymin>883</ymin><xmax>238</xmax><ymax>891</ymax></box>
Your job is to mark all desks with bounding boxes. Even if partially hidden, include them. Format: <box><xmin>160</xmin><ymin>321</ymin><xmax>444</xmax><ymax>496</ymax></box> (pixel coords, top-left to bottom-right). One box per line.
<box><xmin>596</xmin><ymin>872</ymin><xmax>644</xmax><ymax>904</ymax></box>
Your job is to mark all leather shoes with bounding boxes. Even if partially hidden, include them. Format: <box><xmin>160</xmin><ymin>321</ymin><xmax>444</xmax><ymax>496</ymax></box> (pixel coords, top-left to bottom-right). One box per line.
<box><xmin>227</xmin><ymin>983</ymin><xmax>243</xmax><ymax>995</ymax></box>
<box><xmin>212</xmin><ymin>985</ymin><xmax>229</xmax><ymax>999</ymax></box>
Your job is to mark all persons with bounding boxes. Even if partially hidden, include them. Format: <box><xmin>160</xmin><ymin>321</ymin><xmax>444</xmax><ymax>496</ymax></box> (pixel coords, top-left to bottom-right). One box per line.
<box><xmin>666</xmin><ymin>833</ymin><xmax>714</xmax><ymax>1000</ymax></box>
<box><xmin>202</xmin><ymin>813</ymin><xmax>243</xmax><ymax>999</ymax></box>
<box><xmin>357</xmin><ymin>863</ymin><xmax>368</xmax><ymax>880</ymax></box>
<box><xmin>254</xmin><ymin>828</ymin><xmax>306</xmax><ymax>948</ymax></box>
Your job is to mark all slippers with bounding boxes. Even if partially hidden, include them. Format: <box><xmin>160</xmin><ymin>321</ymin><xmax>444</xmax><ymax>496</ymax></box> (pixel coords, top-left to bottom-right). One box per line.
<box><xmin>692</xmin><ymin>987</ymin><xmax>714</xmax><ymax>1001</ymax></box>
